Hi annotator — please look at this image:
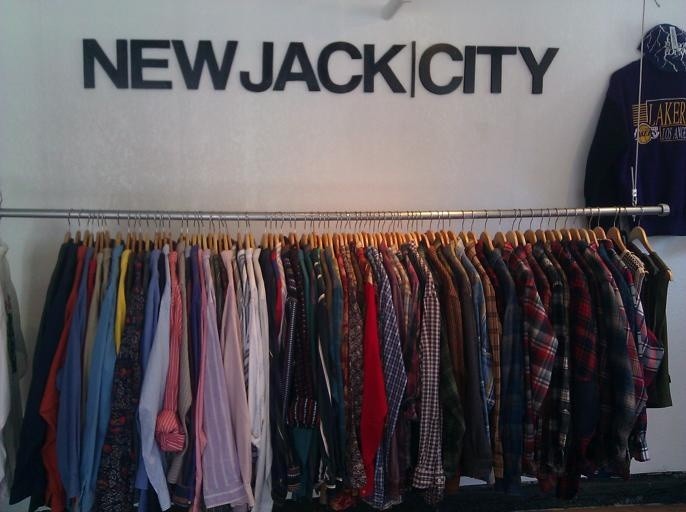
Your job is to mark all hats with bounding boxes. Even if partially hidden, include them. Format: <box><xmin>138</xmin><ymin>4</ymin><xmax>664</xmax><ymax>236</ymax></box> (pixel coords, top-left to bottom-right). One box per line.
<box><xmin>635</xmin><ymin>21</ymin><xmax>685</xmax><ymax>75</ymax></box>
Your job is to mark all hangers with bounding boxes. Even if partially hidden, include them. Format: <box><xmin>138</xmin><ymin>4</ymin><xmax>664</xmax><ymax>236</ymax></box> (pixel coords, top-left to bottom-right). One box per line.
<box><xmin>61</xmin><ymin>207</ymin><xmax>672</xmax><ymax>284</ymax></box>
